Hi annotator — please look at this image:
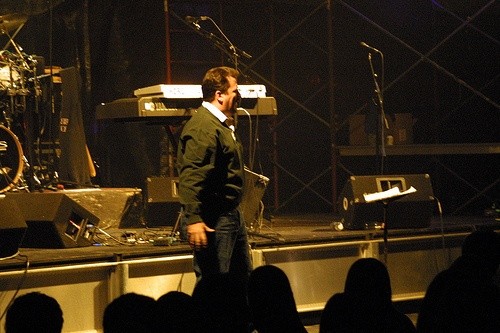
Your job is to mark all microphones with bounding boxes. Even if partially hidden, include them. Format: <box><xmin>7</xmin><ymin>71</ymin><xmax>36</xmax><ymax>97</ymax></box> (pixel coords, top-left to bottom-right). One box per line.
<box><xmin>185</xmin><ymin>16</ymin><xmax>210</xmax><ymax>24</ymax></box>
<box><xmin>360</xmin><ymin>42</ymin><xmax>382</xmax><ymax>54</ymax></box>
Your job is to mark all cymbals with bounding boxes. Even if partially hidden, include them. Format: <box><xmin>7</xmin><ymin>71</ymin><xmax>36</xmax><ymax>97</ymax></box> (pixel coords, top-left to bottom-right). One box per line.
<box><xmin>0</xmin><ymin>12</ymin><xmax>27</xmax><ymax>34</ymax></box>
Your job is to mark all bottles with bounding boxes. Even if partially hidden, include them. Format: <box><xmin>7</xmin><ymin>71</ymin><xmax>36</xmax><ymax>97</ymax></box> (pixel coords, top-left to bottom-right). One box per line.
<box><xmin>330</xmin><ymin>221</ymin><xmax>344</xmax><ymax>232</ymax></box>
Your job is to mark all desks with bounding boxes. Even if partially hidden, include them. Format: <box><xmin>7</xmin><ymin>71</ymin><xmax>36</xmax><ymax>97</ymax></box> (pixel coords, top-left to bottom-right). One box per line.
<box><xmin>338</xmin><ymin>143</ymin><xmax>500</xmax><ymax>217</ymax></box>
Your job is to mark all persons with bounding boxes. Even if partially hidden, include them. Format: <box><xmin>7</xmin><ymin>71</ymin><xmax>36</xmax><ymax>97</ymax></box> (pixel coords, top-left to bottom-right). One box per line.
<box><xmin>5</xmin><ymin>227</ymin><xmax>500</xmax><ymax>333</ymax></box>
<box><xmin>176</xmin><ymin>66</ymin><xmax>253</xmax><ymax>281</ymax></box>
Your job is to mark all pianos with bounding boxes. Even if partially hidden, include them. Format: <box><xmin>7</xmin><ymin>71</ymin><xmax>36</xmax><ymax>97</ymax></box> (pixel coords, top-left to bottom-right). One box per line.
<box><xmin>94</xmin><ymin>81</ymin><xmax>277</xmax><ymax>175</ymax></box>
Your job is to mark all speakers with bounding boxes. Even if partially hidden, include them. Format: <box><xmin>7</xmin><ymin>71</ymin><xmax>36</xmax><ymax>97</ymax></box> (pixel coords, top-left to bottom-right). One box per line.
<box><xmin>142</xmin><ymin>176</ymin><xmax>185</xmax><ymax>227</ymax></box>
<box><xmin>0</xmin><ymin>191</ymin><xmax>100</xmax><ymax>258</ymax></box>
<box><xmin>338</xmin><ymin>173</ymin><xmax>435</xmax><ymax>230</ymax></box>
<box><xmin>238</xmin><ymin>166</ymin><xmax>271</xmax><ymax>228</ymax></box>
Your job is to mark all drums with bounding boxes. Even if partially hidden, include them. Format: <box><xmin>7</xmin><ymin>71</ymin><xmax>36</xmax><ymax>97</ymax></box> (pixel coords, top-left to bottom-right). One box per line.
<box><xmin>0</xmin><ymin>126</ymin><xmax>24</xmax><ymax>193</ymax></box>
